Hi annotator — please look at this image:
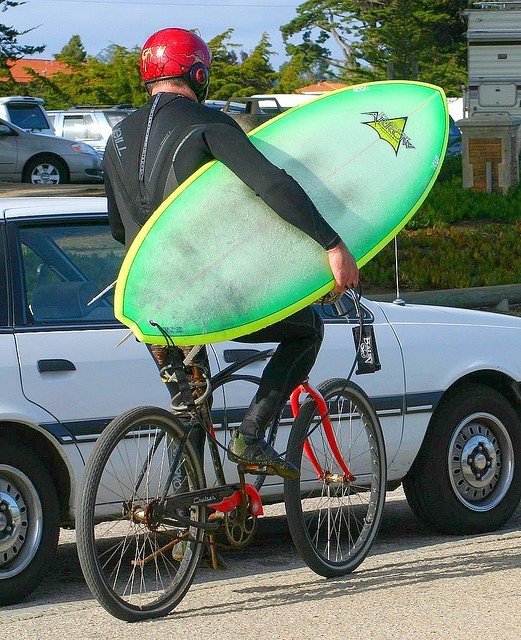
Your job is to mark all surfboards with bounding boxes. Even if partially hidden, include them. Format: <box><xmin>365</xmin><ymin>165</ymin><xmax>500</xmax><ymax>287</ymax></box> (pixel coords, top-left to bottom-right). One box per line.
<box><xmin>114</xmin><ymin>80</ymin><xmax>449</xmax><ymax>346</ymax></box>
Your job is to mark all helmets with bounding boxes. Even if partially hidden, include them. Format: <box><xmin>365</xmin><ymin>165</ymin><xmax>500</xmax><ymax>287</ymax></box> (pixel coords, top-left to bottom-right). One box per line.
<box><xmin>139</xmin><ymin>28</ymin><xmax>211</xmax><ymax>102</ymax></box>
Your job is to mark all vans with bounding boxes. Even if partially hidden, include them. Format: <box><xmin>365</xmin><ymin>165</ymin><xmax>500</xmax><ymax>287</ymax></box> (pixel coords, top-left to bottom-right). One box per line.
<box><xmin>251</xmin><ymin>94</ymin><xmax>319</xmax><ymax>113</ymax></box>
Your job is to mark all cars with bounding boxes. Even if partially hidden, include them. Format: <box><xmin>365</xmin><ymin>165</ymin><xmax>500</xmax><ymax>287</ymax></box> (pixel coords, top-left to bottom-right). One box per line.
<box><xmin>49</xmin><ymin>110</ymin><xmax>128</xmax><ymax>153</ymax></box>
<box><xmin>1</xmin><ymin>194</ymin><xmax>521</xmax><ymax>606</ymax></box>
<box><xmin>0</xmin><ymin>118</ymin><xmax>105</xmax><ymax>184</ymax></box>
<box><xmin>205</xmin><ymin>100</ymin><xmax>266</xmax><ymax>115</ymax></box>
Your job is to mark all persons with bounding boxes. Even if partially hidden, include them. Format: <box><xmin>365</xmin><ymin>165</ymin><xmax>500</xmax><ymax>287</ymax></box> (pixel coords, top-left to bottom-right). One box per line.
<box><xmin>101</xmin><ymin>29</ymin><xmax>358</xmax><ymax>568</ymax></box>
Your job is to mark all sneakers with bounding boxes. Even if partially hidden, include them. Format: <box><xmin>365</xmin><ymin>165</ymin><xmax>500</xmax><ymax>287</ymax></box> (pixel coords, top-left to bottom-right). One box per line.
<box><xmin>173</xmin><ymin>531</ymin><xmax>229</xmax><ymax>573</ymax></box>
<box><xmin>227</xmin><ymin>427</ymin><xmax>301</xmax><ymax>481</ymax></box>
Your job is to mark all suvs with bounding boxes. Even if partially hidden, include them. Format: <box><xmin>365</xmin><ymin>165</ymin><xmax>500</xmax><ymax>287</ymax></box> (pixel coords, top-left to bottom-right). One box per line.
<box><xmin>0</xmin><ymin>96</ymin><xmax>55</xmax><ymax>134</ymax></box>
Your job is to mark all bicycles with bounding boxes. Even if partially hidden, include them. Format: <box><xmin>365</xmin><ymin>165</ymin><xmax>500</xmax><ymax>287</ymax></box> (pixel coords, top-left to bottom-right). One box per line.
<box><xmin>76</xmin><ymin>283</ymin><xmax>388</xmax><ymax>623</ymax></box>
